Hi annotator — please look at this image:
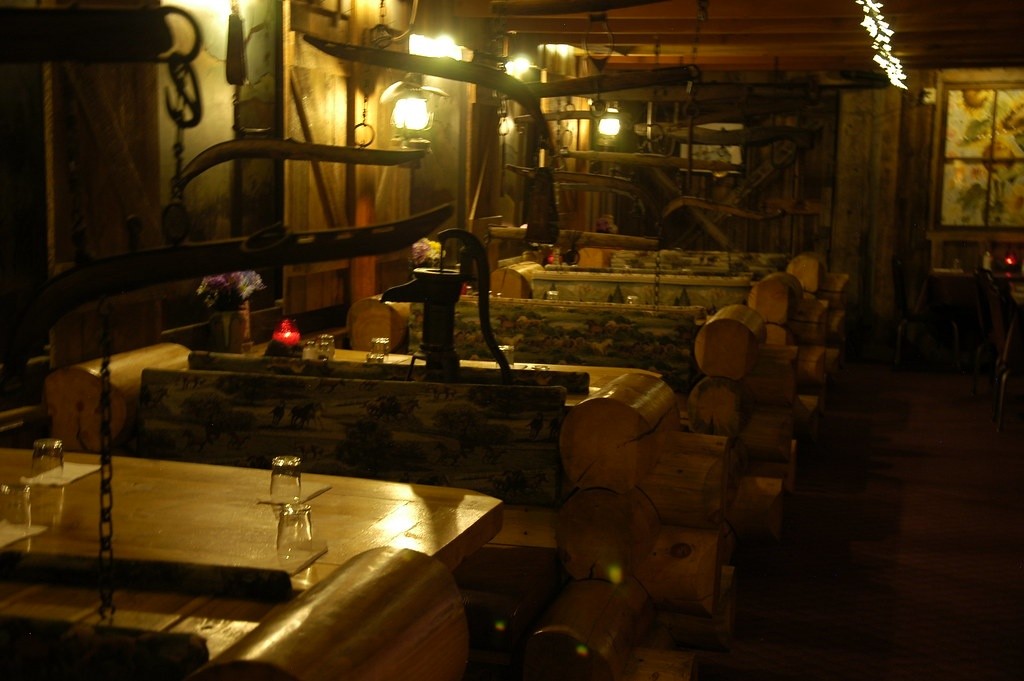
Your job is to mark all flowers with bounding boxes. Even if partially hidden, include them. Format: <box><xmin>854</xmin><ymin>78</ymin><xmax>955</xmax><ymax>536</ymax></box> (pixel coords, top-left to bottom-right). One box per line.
<box><xmin>195</xmin><ymin>268</ymin><xmax>269</xmax><ymax>310</ymax></box>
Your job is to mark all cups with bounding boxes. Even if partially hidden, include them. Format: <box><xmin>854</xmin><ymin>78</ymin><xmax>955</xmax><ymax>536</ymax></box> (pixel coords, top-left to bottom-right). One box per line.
<box><xmin>627</xmin><ymin>295</ymin><xmax>642</xmax><ymax>305</ymax></box>
<box><xmin>367</xmin><ymin>337</ymin><xmax>390</xmax><ymax>362</ymax></box>
<box><xmin>496</xmin><ymin>345</ymin><xmax>514</xmax><ymax>369</ymax></box>
<box><xmin>31</xmin><ymin>438</ymin><xmax>63</xmax><ymax>479</ymax></box>
<box><xmin>1</xmin><ymin>484</ymin><xmax>32</xmax><ymax>531</ymax></box>
<box><xmin>546</xmin><ymin>292</ymin><xmax>560</xmax><ymax>300</ymax></box>
<box><xmin>277</xmin><ymin>504</ymin><xmax>312</xmax><ymax>563</ymax></box>
<box><xmin>270</xmin><ymin>456</ymin><xmax>301</xmax><ymax>506</ymax></box>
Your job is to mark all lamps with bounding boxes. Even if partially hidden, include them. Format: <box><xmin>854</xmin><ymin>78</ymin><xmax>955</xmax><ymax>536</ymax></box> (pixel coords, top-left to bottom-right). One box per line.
<box><xmin>384</xmin><ymin>83</ymin><xmax>446</xmax><ymax>147</ymax></box>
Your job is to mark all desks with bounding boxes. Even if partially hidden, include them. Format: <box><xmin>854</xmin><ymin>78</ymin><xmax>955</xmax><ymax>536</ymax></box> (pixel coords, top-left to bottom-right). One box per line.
<box><xmin>1</xmin><ymin>447</ymin><xmax>504</xmax><ymax>610</ymax></box>
<box><xmin>914</xmin><ymin>266</ymin><xmax>1021</xmax><ymax>368</ymax></box>
<box><xmin>266</xmin><ymin>347</ymin><xmax>672</xmax><ymax>406</ymax></box>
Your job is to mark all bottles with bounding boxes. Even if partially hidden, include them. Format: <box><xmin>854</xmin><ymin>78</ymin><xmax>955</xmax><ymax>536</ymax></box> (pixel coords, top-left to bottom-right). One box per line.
<box><xmin>528</xmin><ymin>243</ymin><xmax>562</xmax><ymax>267</ymax></box>
<box><xmin>303</xmin><ymin>333</ymin><xmax>336</xmax><ymax>361</ymax></box>
<box><xmin>548</xmin><ymin>98</ymin><xmax>575</xmax><ymax>111</ymax></box>
<box><xmin>533</xmin><ymin>121</ymin><xmax>572</xmax><ymax>171</ymax></box>
<box><xmin>673</xmin><ymin>98</ymin><xmax>688</xmax><ymax>126</ymax></box>
<box><xmin>462</xmin><ymin>282</ymin><xmax>503</xmax><ymax>298</ymax></box>
<box><xmin>711</xmin><ymin>146</ymin><xmax>731</xmax><ymax>176</ymax></box>
<box><xmin>596</xmin><ymin>214</ymin><xmax>618</xmax><ymax>233</ymax></box>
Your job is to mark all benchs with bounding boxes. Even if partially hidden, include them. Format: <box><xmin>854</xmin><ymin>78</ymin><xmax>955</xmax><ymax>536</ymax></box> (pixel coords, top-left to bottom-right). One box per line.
<box><xmin>1</xmin><ymin>248</ymin><xmax>851</xmax><ymax>680</ymax></box>
<box><xmin>125</xmin><ymin>249</ymin><xmax>790</xmax><ymax>587</ymax></box>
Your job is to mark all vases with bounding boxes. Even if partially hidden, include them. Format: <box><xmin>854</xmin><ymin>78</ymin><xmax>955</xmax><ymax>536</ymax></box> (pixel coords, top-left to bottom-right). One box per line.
<box><xmin>210</xmin><ymin>299</ymin><xmax>252</xmax><ymax>353</ymax></box>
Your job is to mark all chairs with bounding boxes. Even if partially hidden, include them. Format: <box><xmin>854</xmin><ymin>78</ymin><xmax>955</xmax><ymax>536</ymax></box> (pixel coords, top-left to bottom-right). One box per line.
<box><xmin>971</xmin><ymin>268</ymin><xmax>1024</xmax><ymax>423</ymax></box>
<box><xmin>892</xmin><ymin>235</ymin><xmax>969</xmax><ymax>369</ymax></box>
